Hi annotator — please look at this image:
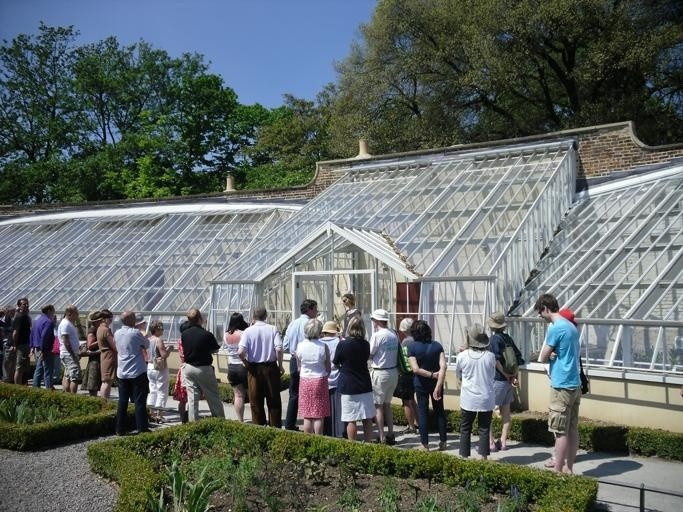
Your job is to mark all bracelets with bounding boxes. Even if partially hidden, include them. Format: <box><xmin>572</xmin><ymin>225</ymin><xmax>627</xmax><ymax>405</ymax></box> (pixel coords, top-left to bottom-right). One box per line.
<box><xmin>431</xmin><ymin>372</ymin><xmax>433</xmax><ymax>379</ymax></box>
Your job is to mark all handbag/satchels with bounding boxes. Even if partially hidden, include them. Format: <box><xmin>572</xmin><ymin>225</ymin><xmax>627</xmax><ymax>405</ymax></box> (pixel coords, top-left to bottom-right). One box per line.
<box><xmin>153</xmin><ymin>353</ymin><xmax>167</xmax><ymax>371</ymax></box>
<box><xmin>578</xmin><ymin>367</ymin><xmax>591</xmax><ymax>394</ymax></box>
<box><xmin>50</xmin><ymin>335</ymin><xmax>60</xmax><ymax>354</ymax></box>
<box><xmin>390</xmin><ymin>327</ymin><xmax>413</xmax><ymax>376</ymax></box>
<box><xmin>76</xmin><ymin>327</ymin><xmax>101</xmax><ymax>357</ymax></box>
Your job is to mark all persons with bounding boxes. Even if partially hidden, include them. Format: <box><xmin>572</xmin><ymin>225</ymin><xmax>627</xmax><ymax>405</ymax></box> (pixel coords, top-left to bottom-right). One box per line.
<box><xmin>319</xmin><ymin>296</ymin><xmax>362</xmax><ymax>438</ymax></box>
<box><xmin>113</xmin><ymin>311</ymin><xmax>153</xmax><ymax>435</ymax></box>
<box><xmin>172</xmin><ymin>309</ymin><xmax>225</xmax><ymax>424</ymax></box>
<box><xmin>223</xmin><ymin>312</ymin><xmax>249</xmax><ymax>421</ymax></box>
<box><xmin>399</xmin><ymin>318</ymin><xmax>420</xmax><ymax>435</ymax></box>
<box><xmin>486</xmin><ymin>312</ymin><xmax>525</xmax><ymax>452</ymax></box>
<box><xmin>333</xmin><ymin>315</ymin><xmax>376</xmax><ymax>443</ymax></box>
<box><xmin>407</xmin><ymin>320</ymin><xmax>447</xmax><ymax>451</ymax></box>
<box><xmin>2</xmin><ymin>298</ymin><xmax>118</xmax><ymax>399</ymax></box>
<box><xmin>283</xmin><ymin>299</ymin><xmax>318</xmax><ymax>431</ymax></box>
<box><xmin>237</xmin><ymin>306</ymin><xmax>285</xmax><ymax>428</ymax></box>
<box><xmin>135</xmin><ymin>314</ymin><xmax>175</xmax><ymax>424</ymax></box>
<box><xmin>534</xmin><ymin>296</ymin><xmax>582</xmax><ymax>471</ymax></box>
<box><xmin>295</xmin><ymin>318</ymin><xmax>331</xmax><ymax>435</ymax></box>
<box><xmin>455</xmin><ymin>324</ymin><xmax>497</xmax><ymax>461</ymax></box>
<box><xmin>370</xmin><ymin>309</ymin><xmax>400</xmax><ymax>445</ymax></box>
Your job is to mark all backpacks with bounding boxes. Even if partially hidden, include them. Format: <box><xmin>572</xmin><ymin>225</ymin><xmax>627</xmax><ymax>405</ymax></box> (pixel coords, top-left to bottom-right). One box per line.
<box><xmin>496</xmin><ymin>333</ymin><xmax>519</xmax><ymax>376</ymax></box>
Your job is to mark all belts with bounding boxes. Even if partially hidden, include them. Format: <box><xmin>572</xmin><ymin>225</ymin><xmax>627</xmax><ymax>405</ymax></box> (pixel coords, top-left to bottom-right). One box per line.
<box><xmin>372</xmin><ymin>364</ymin><xmax>400</xmax><ymax>371</ymax></box>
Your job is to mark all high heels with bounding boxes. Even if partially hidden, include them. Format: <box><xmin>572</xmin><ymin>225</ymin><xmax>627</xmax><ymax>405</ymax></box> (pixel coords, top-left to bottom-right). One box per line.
<box><xmin>149</xmin><ymin>414</ymin><xmax>170</xmax><ymax>424</ymax></box>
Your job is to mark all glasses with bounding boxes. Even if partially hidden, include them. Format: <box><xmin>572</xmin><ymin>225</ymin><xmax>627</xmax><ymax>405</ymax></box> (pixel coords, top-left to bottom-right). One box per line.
<box><xmin>537</xmin><ymin>308</ymin><xmax>545</xmax><ymax>315</ymax></box>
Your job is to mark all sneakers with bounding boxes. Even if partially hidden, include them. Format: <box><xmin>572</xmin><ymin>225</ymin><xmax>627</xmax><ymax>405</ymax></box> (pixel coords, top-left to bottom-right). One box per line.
<box><xmin>543</xmin><ymin>454</ymin><xmax>566</xmax><ymax>467</ymax></box>
<box><xmin>473</xmin><ymin>443</ymin><xmax>498</xmax><ymax>453</ymax></box>
<box><xmin>397</xmin><ymin>424</ymin><xmax>419</xmax><ymax>435</ymax></box>
<box><xmin>285</xmin><ymin>426</ymin><xmax>300</xmax><ymax>430</ymax></box>
<box><xmin>137</xmin><ymin>429</ymin><xmax>152</xmax><ymax>434</ymax></box>
<box><xmin>494</xmin><ymin>438</ymin><xmax>508</xmax><ymax>451</ymax></box>
<box><xmin>437</xmin><ymin>441</ymin><xmax>447</xmax><ymax>451</ymax></box>
<box><xmin>410</xmin><ymin>444</ymin><xmax>431</xmax><ymax>453</ymax></box>
<box><xmin>371</xmin><ymin>434</ymin><xmax>396</xmax><ymax>447</ymax></box>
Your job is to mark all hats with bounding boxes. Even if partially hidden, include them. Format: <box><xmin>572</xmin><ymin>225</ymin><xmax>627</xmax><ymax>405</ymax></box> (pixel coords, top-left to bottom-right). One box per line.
<box><xmin>87</xmin><ymin>310</ymin><xmax>104</xmax><ymax>323</ymax></box>
<box><xmin>486</xmin><ymin>309</ymin><xmax>508</xmax><ymax>330</ymax></box>
<box><xmin>133</xmin><ymin>311</ymin><xmax>146</xmax><ymax>327</ymax></box>
<box><xmin>368</xmin><ymin>308</ymin><xmax>390</xmax><ymax>322</ymax></box>
<box><xmin>558</xmin><ymin>307</ymin><xmax>579</xmax><ymax>327</ymax></box>
<box><xmin>397</xmin><ymin>317</ymin><xmax>415</xmax><ymax>332</ymax></box>
<box><xmin>4</xmin><ymin>305</ymin><xmax>16</xmax><ymax>312</ymax></box>
<box><xmin>464</xmin><ymin>322</ymin><xmax>491</xmax><ymax>350</ymax></box>
<box><xmin>321</xmin><ymin>320</ymin><xmax>338</xmax><ymax>334</ymax></box>
